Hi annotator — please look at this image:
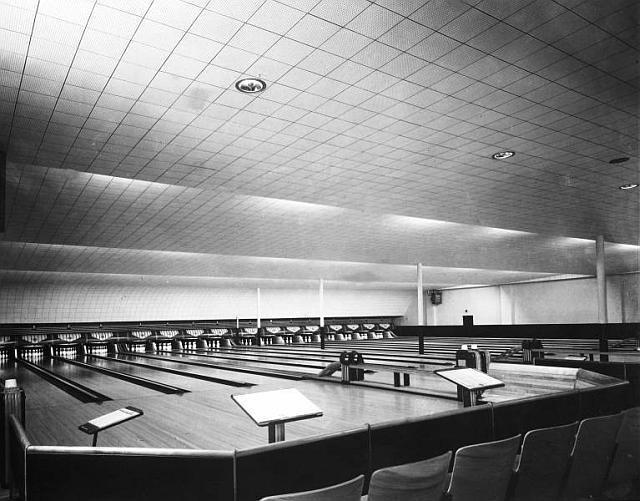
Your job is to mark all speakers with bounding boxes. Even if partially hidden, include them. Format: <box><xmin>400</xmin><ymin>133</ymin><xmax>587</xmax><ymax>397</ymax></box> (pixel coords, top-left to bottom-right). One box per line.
<box><xmin>431</xmin><ymin>292</ymin><xmax>442</xmax><ymax>304</ymax></box>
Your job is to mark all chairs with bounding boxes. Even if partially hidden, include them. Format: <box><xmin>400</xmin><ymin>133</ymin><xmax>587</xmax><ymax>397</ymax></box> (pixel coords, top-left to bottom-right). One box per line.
<box><xmin>445</xmin><ymin>434</ymin><xmax>521</xmax><ymax>500</ymax></box>
<box><xmin>259</xmin><ymin>475</ymin><xmax>364</xmax><ymax>501</ymax></box>
<box><xmin>362</xmin><ymin>451</ymin><xmax>452</xmax><ymax>500</ymax></box>
<box><xmin>510</xmin><ymin>421</ymin><xmax>578</xmax><ymax>500</ymax></box>
<box><xmin>600</xmin><ymin>407</ymin><xmax>639</xmax><ymax>499</ymax></box>
<box><xmin>566</xmin><ymin>412</ymin><xmax>620</xmax><ymax>500</ymax></box>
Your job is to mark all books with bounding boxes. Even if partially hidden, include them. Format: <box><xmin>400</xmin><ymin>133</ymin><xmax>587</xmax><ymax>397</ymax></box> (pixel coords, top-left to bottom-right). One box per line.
<box><xmin>87</xmin><ymin>408</ymin><xmax>140</xmax><ymax>429</ymax></box>
<box><xmin>232</xmin><ymin>388</ymin><xmax>324</xmax><ymax>427</ymax></box>
<box><xmin>436</xmin><ymin>368</ymin><xmax>505</xmax><ymax>391</ymax></box>
